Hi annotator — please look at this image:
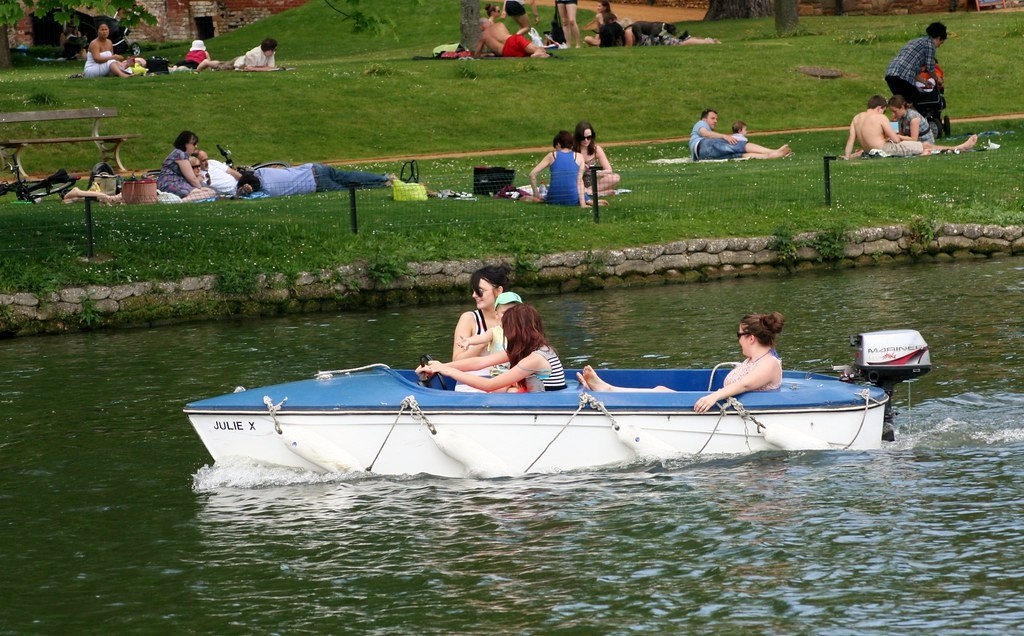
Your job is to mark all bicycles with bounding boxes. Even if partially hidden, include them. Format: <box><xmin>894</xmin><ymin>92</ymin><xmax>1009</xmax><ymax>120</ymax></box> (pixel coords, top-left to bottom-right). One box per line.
<box><xmin>87</xmin><ymin>162</ymin><xmax>139</xmax><ymax>195</ymax></box>
<box><xmin>140</xmin><ymin>143</ymin><xmax>291</xmax><ymax>182</ymax></box>
<box><xmin>0</xmin><ymin>154</ymin><xmax>82</xmax><ymax>205</ymax></box>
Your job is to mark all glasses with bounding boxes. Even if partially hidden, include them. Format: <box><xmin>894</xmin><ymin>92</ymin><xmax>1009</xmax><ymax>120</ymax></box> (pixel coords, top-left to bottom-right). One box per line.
<box><xmin>471</xmin><ymin>288</ymin><xmax>486</xmax><ymax>298</ymax></box>
<box><xmin>192</xmin><ymin>164</ymin><xmax>200</xmax><ymax>169</ymax></box>
<box><xmin>206</xmin><ymin>173</ymin><xmax>211</xmax><ymax>185</ymax></box>
<box><xmin>583</xmin><ymin>135</ymin><xmax>593</xmax><ymax>140</ymax></box>
<box><xmin>193</xmin><ymin>142</ymin><xmax>197</xmax><ymax>146</ymax></box>
<box><xmin>736</xmin><ymin>331</ymin><xmax>751</xmax><ymax>339</ymax></box>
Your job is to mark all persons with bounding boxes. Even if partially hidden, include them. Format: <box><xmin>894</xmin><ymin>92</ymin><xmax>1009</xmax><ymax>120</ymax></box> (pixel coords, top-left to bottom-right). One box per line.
<box><xmin>689</xmin><ymin>109</ymin><xmax>794</xmax><ymax>160</ymax></box>
<box><xmin>169</xmin><ymin>40</ymin><xmax>210</xmax><ymax>71</ymax></box>
<box><xmin>840</xmin><ymin>20</ymin><xmax>978</xmax><ymax>161</ymax></box>
<box><xmin>85</xmin><ymin>22</ymin><xmax>133</xmax><ymax>78</ymax></box>
<box><xmin>577</xmin><ymin>310</ymin><xmax>786</xmax><ymax>413</ymax></box>
<box><xmin>474</xmin><ymin>0</ymin><xmax>723</xmax><ymax>58</ymax></box>
<box><xmin>198</xmin><ymin>38</ymin><xmax>277</xmax><ymax>71</ymax></box>
<box><xmin>64</xmin><ymin>131</ymin><xmax>400</xmax><ymax>204</ymax></box>
<box><xmin>415</xmin><ymin>262</ymin><xmax>567</xmax><ymax>395</ymax></box>
<box><xmin>521</xmin><ymin>122</ymin><xmax>619</xmax><ymax>209</ymax></box>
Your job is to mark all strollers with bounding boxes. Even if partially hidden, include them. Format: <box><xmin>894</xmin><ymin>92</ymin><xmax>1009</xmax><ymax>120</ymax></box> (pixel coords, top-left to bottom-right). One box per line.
<box><xmin>94</xmin><ymin>3</ymin><xmax>141</xmax><ymax>56</ymax></box>
<box><xmin>913</xmin><ymin>56</ymin><xmax>951</xmax><ymax>139</ymax></box>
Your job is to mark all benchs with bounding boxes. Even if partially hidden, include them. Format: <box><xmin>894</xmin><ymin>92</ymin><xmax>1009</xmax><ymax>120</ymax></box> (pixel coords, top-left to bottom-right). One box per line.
<box><xmin>546</xmin><ymin>379</ymin><xmax>590</xmax><ymax>393</ymax></box>
<box><xmin>0</xmin><ymin>108</ymin><xmax>141</xmax><ymax>178</ymax></box>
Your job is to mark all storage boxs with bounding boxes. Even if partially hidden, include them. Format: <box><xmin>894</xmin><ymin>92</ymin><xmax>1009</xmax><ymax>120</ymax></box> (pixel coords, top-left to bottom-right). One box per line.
<box><xmin>473</xmin><ymin>167</ymin><xmax>515</xmax><ymax>195</ymax></box>
<box><xmin>121</xmin><ymin>180</ymin><xmax>158</xmax><ymax>204</ymax></box>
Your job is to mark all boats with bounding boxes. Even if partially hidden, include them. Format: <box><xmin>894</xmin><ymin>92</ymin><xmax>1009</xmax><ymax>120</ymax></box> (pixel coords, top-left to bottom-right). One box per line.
<box><xmin>181</xmin><ymin>327</ymin><xmax>934</xmax><ymax>479</ymax></box>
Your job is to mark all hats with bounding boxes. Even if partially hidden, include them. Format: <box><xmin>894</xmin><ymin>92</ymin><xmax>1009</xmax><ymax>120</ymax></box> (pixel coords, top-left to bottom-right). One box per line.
<box><xmin>190</xmin><ymin>39</ymin><xmax>207</xmax><ymax>51</ymax></box>
<box><xmin>494</xmin><ymin>292</ymin><xmax>523</xmax><ymax>312</ymax></box>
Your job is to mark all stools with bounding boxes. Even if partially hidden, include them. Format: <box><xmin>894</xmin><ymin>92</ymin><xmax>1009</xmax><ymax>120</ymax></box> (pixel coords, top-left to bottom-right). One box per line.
<box><xmin>966</xmin><ymin>0</ymin><xmax>1007</xmax><ymax>12</ymax></box>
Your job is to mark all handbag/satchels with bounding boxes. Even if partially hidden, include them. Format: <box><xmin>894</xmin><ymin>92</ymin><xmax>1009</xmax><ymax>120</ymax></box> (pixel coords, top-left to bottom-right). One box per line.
<box><xmin>433</xmin><ymin>43</ymin><xmax>469</xmax><ymax>58</ymax></box>
<box><xmin>527</xmin><ymin>27</ymin><xmax>543</xmax><ymax>47</ymax></box>
<box><xmin>473</xmin><ymin>165</ymin><xmax>516</xmax><ymax>195</ymax></box>
<box><xmin>146</xmin><ymin>57</ymin><xmax>170</xmax><ymax>74</ymax></box>
<box><xmin>551</xmin><ymin>20</ymin><xmax>567</xmax><ymax>44</ymax></box>
<box><xmin>393</xmin><ymin>160</ymin><xmax>428</xmax><ymax>202</ymax></box>
